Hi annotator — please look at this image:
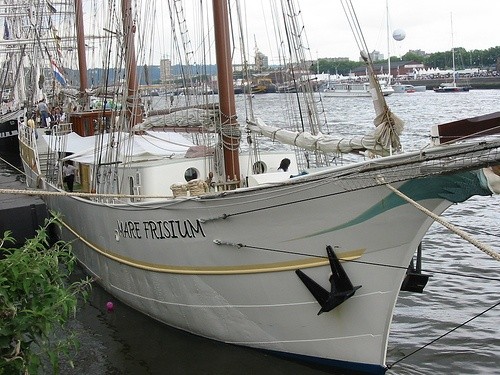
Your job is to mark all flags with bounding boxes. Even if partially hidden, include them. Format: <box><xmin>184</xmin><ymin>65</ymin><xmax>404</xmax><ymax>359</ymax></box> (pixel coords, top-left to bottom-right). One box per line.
<box><xmin>51</xmin><ymin>56</ymin><xmax>67</xmax><ymax>88</ymax></box>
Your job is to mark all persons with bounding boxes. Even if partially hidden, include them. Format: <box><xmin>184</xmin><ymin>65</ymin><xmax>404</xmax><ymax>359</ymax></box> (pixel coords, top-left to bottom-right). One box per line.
<box><xmin>35</xmin><ymin>98</ymin><xmax>110</xmax><ymax>130</ymax></box>
<box><xmin>62</xmin><ymin>160</ymin><xmax>80</xmax><ymax>192</ymax></box>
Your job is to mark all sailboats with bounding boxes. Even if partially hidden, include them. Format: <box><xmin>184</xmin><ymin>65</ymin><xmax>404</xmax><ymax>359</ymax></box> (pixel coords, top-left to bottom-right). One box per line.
<box><xmin>1</xmin><ymin>0</ymin><xmax>500</xmax><ymax>374</ymax></box>
<box><xmin>433</xmin><ymin>13</ymin><xmax>471</xmax><ymax>93</ymax></box>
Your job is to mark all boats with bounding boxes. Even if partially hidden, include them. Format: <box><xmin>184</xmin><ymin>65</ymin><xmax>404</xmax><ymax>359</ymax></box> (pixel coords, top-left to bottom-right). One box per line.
<box><xmin>324</xmin><ymin>82</ymin><xmax>394</xmax><ymax>99</ymax></box>
